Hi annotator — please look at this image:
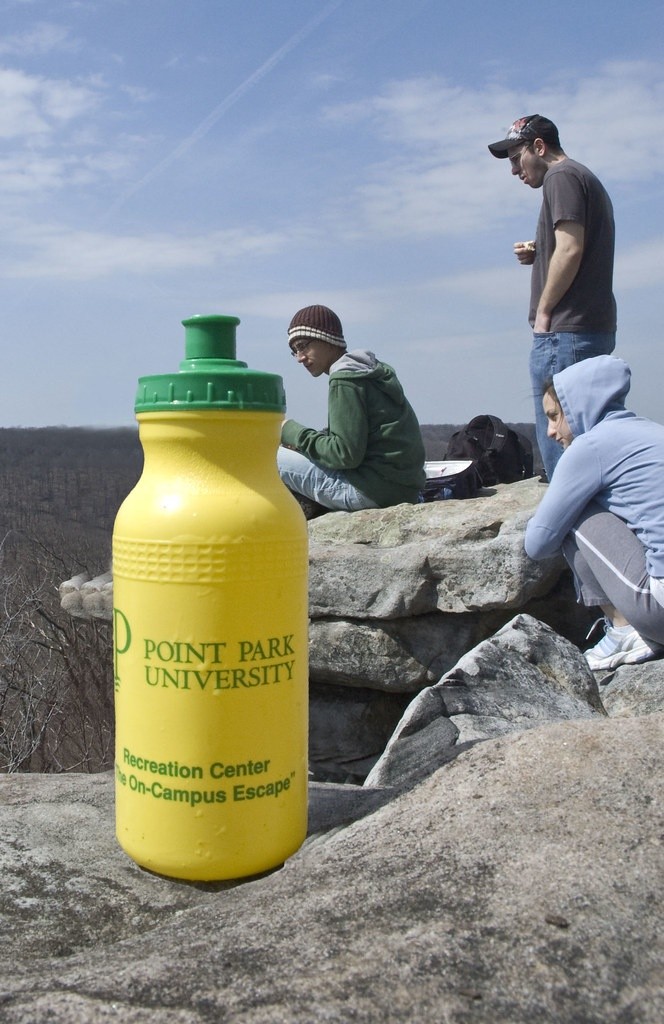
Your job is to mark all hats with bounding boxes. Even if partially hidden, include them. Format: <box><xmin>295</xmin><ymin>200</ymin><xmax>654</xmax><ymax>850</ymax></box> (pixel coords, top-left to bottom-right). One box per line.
<box><xmin>488</xmin><ymin>113</ymin><xmax>560</xmax><ymax>158</ymax></box>
<box><xmin>287</xmin><ymin>305</ymin><xmax>347</xmax><ymax>349</ymax></box>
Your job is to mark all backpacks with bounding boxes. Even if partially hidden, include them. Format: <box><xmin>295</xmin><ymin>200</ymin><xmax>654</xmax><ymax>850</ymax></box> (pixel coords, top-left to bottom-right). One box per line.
<box><xmin>418</xmin><ymin>460</ymin><xmax>484</xmax><ymax>503</ymax></box>
<box><xmin>443</xmin><ymin>415</ymin><xmax>534</xmax><ymax>487</ymax></box>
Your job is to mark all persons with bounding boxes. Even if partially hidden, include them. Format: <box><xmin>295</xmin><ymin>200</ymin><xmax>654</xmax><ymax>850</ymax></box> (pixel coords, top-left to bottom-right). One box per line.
<box><xmin>488</xmin><ymin>113</ymin><xmax>617</xmax><ymax>492</ymax></box>
<box><xmin>525</xmin><ymin>356</ymin><xmax>664</xmax><ymax>671</ymax></box>
<box><xmin>276</xmin><ymin>305</ymin><xmax>426</xmax><ymax>518</ymax></box>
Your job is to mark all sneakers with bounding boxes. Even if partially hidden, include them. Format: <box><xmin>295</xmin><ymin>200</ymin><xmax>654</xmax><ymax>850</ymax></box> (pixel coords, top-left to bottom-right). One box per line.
<box><xmin>583</xmin><ymin>623</ymin><xmax>656</xmax><ymax>671</ymax></box>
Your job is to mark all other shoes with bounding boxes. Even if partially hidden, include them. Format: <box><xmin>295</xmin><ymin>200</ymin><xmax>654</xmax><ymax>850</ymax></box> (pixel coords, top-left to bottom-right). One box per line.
<box><xmin>293</xmin><ymin>493</ymin><xmax>319</xmax><ymax>523</ymax></box>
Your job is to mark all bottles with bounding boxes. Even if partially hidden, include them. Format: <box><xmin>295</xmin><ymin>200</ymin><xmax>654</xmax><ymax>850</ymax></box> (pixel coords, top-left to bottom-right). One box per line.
<box><xmin>105</xmin><ymin>309</ymin><xmax>313</xmax><ymax>881</ymax></box>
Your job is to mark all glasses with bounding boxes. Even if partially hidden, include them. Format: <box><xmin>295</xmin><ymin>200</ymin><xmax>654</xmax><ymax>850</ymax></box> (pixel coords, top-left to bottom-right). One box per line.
<box><xmin>291</xmin><ymin>337</ymin><xmax>318</xmax><ymax>357</ymax></box>
<box><xmin>508</xmin><ymin>141</ymin><xmax>533</xmax><ymax>163</ymax></box>
